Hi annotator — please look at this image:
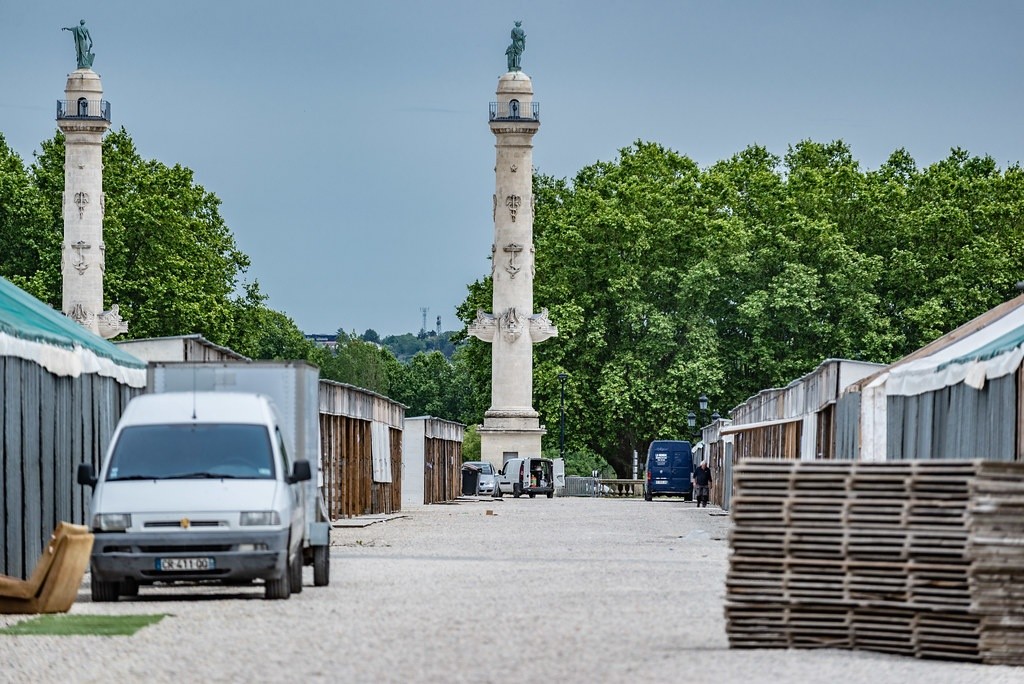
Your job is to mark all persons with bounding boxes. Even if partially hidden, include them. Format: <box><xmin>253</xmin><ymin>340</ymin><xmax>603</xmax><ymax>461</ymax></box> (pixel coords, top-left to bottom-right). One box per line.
<box><xmin>505</xmin><ymin>18</ymin><xmax>526</xmax><ymax>72</ymax></box>
<box><xmin>62</xmin><ymin>19</ymin><xmax>93</xmax><ymax>69</ymax></box>
<box><xmin>693</xmin><ymin>459</ymin><xmax>712</xmax><ymax>506</ymax></box>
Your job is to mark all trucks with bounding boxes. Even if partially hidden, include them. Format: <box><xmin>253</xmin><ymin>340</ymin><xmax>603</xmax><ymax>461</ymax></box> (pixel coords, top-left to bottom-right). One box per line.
<box><xmin>493</xmin><ymin>457</ymin><xmax>565</xmax><ymax>499</ymax></box>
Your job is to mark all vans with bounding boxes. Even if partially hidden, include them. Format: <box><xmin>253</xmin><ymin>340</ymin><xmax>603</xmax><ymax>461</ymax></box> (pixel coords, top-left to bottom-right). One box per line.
<box><xmin>643</xmin><ymin>439</ymin><xmax>693</xmax><ymax>501</ymax></box>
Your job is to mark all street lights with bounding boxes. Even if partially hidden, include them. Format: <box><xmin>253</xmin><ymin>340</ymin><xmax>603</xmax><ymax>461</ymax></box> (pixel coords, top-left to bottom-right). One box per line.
<box><xmin>689</xmin><ymin>393</ymin><xmax>721</xmax><ymax>460</ymax></box>
<box><xmin>557</xmin><ymin>374</ymin><xmax>568</xmax><ymax>457</ymax></box>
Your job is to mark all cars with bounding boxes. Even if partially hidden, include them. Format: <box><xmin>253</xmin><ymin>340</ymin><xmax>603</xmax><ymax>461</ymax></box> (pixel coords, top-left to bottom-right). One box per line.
<box><xmin>77</xmin><ymin>390</ymin><xmax>311</xmax><ymax>601</ymax></box>
<box><xmin>463</xmin><ymin>461</ymin><xmax>497</xmax><ymax>495</ymax></box>
